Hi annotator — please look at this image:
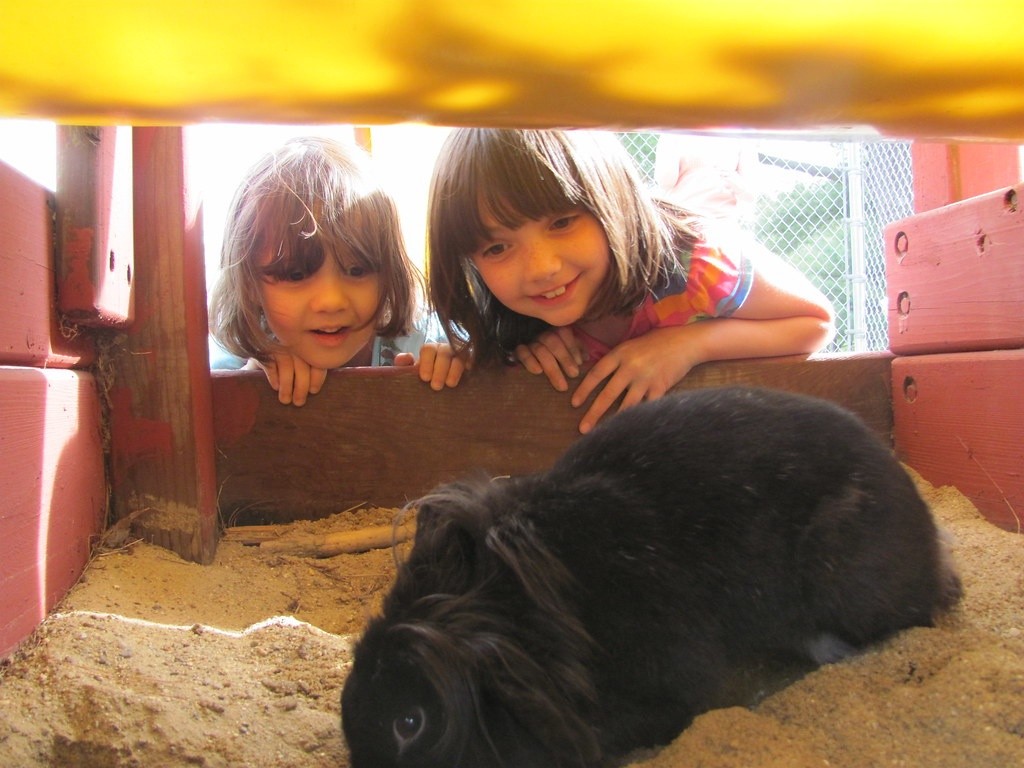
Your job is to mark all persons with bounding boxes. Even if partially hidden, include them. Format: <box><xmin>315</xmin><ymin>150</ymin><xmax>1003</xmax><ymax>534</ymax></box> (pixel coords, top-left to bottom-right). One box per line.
<box><xmin>209</xmin><ymin>137</ymin><xmax>475</xmax><ymax>407</ymax></box>
<box><xmin>427</xmin><ymin>127</ymin><xmax>837</xmax><ymax>436</ymax></box>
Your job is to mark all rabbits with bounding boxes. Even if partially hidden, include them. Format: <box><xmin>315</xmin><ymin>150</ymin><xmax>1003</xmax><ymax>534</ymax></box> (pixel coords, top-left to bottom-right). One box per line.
<box><xmin>340</xmin><ymin>385</ymin><xmax>965</xmax><ymax>768</ymax></box>
